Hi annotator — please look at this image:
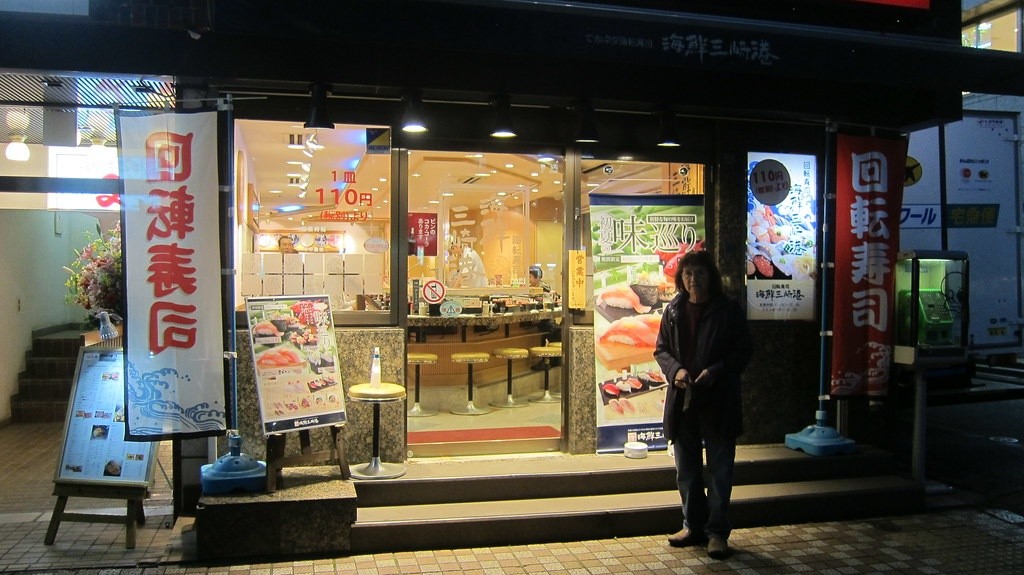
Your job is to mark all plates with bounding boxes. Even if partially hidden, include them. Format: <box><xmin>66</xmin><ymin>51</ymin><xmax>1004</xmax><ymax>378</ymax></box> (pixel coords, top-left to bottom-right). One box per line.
<box><xmin>439</xmin><ymin>301</ymin><xmax>464</xmax><ymax>317</ymax></box>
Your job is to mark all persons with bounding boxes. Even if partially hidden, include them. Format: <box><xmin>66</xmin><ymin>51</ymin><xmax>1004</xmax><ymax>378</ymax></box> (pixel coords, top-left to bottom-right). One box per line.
<box><xmin>449</xmin><ymin>242</ymin><xmax>487</xmax><ymax>287</ymax></box>
<box><xmin>654</xmin><ymin>251</ymin><xmax>752</xmax><ymax>558</ymax></box>
<box><xmin>529</xmin><ymin>266</ymin><xmax>551</xmax><ymax>292</ymax></box>
<box><xmin>531</xmin><ymin>321</ymin><xmax>562</xmax><ymax>370</ymax></box>
<box><xmin>278</xmin><ymin>237</ymin><xmax>304</xmax><ymax>288</ymax></box>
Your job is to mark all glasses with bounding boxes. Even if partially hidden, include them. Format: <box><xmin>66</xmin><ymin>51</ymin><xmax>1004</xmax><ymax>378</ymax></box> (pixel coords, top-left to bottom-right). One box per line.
<box><xmin>679</xmin><ymin>269</ymin><xmax>707</xmax><ymax>278</ymax></box>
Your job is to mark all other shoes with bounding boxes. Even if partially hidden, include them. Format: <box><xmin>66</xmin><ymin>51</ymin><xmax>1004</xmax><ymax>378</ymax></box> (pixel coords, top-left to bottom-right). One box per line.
<box><xmin>668</xmin><ymin>526</ymin><xmax>703</xmax><ymax>546</ymax></box>
<box><xmin>707</xmin><ymin>536</ymin><xmax>728</xmax><ymax>557</ymax></box>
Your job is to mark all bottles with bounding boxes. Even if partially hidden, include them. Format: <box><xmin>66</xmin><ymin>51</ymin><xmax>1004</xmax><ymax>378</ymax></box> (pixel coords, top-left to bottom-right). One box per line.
<box><xmin>369</xmin><ymin>346</ymin><xmax>382</xmax><ymax>388</ymax></box>
<box><xmin>482</xmin><ymin>300</ymin><xmax>489</xmax><ymax>316</ymax></box>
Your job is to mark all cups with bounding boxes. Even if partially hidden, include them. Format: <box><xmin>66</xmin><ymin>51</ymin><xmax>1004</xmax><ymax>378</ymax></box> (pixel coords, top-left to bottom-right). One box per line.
<box><xmin>543</xmin><ymin>293</ymin><xmax>555</xmax><ymax>312</ymax></box>
<box><xmin>419</xmin><ymin>306</ymin><xmax>427</xmax><ymax>315</ymax></box>
<box><xmin>357</xmin><ymin>294</ymin><xmax>366</xmax><ymax>310</ymax></box>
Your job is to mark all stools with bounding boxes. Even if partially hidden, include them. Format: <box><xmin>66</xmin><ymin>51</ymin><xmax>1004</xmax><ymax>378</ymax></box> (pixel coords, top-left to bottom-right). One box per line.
<box><xmin>528</xmin><ymin>346</ymin><xmax>562</xmax><ymax>404</ymax></box>
<box><xmin>450</xmin><ymin>352</ymin><xmax>492</xmax><ymax>415</ymax></box>
<box><xmin>406</xmin><ymin>353</ymin><xmax>438</xmax><ymax>417</ymax></box>
<box><xmin>347</xmin><ymin>382</ymin><xmax>407</xmax><ymax>480</ymax></box>
<box><xmin>488</xmin><ymin>348</ymin><xmax>528</xmax><ymax>408</ymax></box>
<box><xmin>548</xmin><ymin>342</ymin><xmax>562</xmax><ymax>348</ymax></box>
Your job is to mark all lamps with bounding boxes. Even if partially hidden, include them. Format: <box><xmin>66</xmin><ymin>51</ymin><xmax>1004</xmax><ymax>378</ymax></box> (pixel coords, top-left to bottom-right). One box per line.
<box><xmin>5</xmin><ymin>133</ymin><xmax>30</xmax><ymax>161</ymax></box>
<box><xmin>297</xmin><ymin>130</ymin><xmax>318</xmax><ymax>200</ymax></box>
<box><xmin>99</xmin><ymin>311</ymin><xmax>123</xmax><ymax>340</ymax></box>
<box><xmin>304</xmin><ymin>82</ymin><xmax>684</xmax><ymax>148</ymax></box>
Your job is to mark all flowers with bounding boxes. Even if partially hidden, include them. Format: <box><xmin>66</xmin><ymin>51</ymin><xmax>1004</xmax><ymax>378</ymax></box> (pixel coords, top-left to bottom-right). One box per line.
<box><xmin>61</xmin><ymin>219</ymin><xmax>123</xmax><ymax>330</ymax></box>
